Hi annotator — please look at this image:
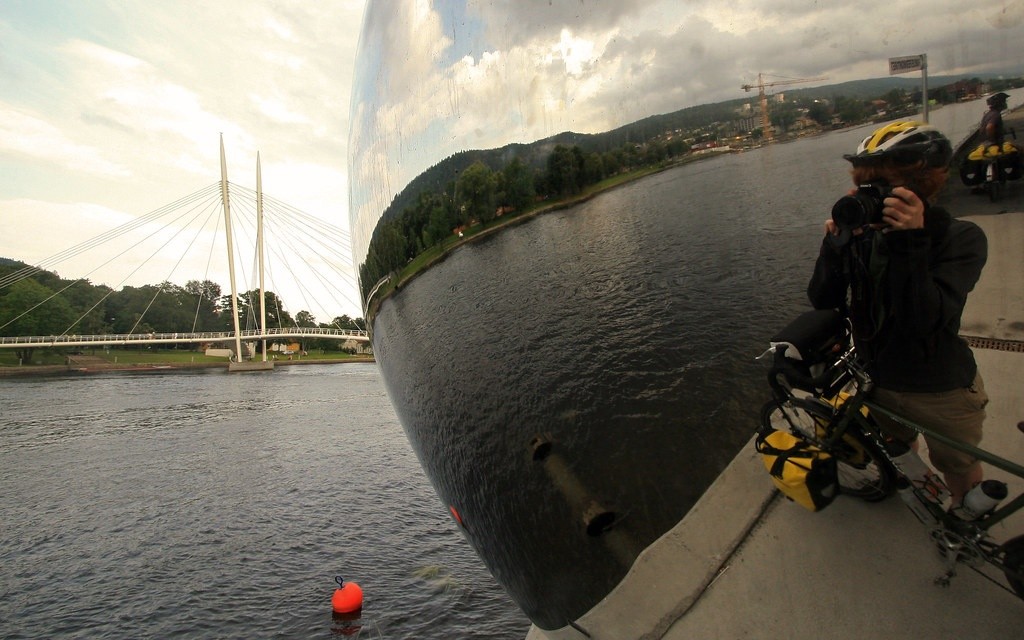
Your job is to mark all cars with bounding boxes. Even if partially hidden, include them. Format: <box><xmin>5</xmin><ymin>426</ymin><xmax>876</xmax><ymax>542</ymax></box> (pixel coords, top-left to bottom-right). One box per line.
<box><xmin>282</xmin><ymin>350</ymin><xmax>294</xmax><ymax>355</ymax></box>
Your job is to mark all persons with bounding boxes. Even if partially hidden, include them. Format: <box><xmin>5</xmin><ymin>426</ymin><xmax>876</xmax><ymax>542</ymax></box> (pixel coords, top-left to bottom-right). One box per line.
<box><xmin>806</xmin><ymin>121</ymin><xmax>989</xmax><ymax>547</ymax></box>
<box><xmin>980</xmin><ymin>92</ymin><xmax>1011</xmax><ymax>195</ymax></box>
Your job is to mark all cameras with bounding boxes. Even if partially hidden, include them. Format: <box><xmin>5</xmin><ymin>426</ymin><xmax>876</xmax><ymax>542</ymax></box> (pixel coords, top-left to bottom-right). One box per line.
<box><xmin>831</xmin><ymin>176</ymin><xmax>909</xmax><ymax>231</ymax></box>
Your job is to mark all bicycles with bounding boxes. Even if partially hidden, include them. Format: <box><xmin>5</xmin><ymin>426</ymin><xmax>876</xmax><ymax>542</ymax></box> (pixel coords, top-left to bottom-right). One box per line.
<box><xmin>756</xmin><ymin>302</ymin><xmax>1024</xmax><ymax>600</ymax></box>
<box><xmin>980</xmin><ymin>130</ymin><xmax>1016</xmax><ymax>202</ymax></box>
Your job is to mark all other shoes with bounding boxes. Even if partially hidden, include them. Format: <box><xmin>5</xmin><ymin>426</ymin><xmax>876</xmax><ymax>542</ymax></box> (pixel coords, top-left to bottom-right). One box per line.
<box><xmin>859</xmin><ymin>472</ymin><xmax>912</xmax><ymax>492</ymax></box>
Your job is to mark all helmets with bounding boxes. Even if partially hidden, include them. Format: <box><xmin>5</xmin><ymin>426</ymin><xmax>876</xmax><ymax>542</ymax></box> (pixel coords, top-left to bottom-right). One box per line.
<box><xmin>986</xmin><ymin>93</ymin><xmax>1010</xmax><ymax>105</ymax></box>
<box><xmin>843</xmin><ymin>118</ymin><xmax>951</xmax><ymax>172</ymax></box>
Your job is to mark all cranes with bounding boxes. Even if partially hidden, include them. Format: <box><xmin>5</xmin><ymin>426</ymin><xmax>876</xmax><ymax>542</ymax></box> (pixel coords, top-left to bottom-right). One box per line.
<box><xmin>740</xmin><ymin>74</ymin><xmax>823</xmax><ymax>140</ymax></box>
